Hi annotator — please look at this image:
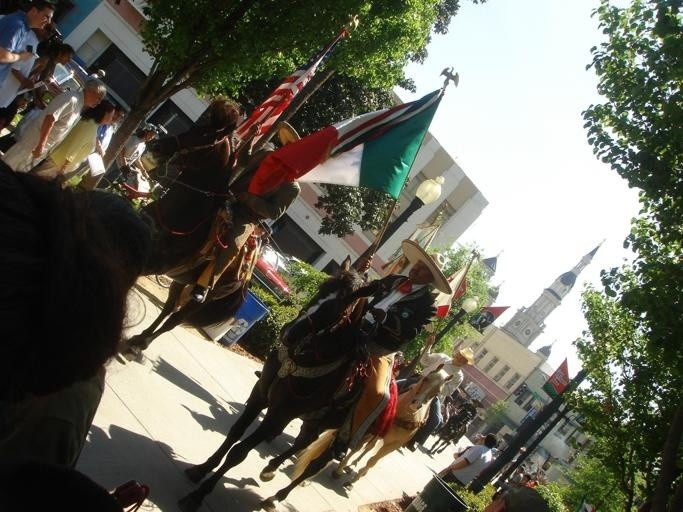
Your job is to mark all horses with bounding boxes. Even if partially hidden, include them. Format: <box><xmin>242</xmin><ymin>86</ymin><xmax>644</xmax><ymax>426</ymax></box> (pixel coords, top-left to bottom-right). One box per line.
<box><xmin>176</xmin><ymin>254</ymin><xmax>400</xmax><ymax>512</ymax></box>
<box><xmin>119</xmin><ymin>94</ymin><xmax>254</xmax><ymax>362</ymax></box>
<box><xmin>331</xmin><ymin>364</ymin><xmax>456</xmax><ymax>494</ymax></box>
<box><xmin>426</xmin><ymin>408</ymin><xmax>468</xmax><ymax>458</ymax></box>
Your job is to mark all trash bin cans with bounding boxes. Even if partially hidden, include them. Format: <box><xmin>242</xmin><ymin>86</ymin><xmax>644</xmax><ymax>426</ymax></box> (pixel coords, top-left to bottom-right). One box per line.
<box><xmin>218</xmin><ymin>290</ymin><xmax>270</xmax><ymax>348</ymax></box>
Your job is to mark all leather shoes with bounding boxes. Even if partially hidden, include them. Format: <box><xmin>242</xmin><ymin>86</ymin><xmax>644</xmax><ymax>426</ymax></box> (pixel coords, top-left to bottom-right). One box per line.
<box><xmin>330</xmin><ymin>438</ymin><xmax>348</xmax><ymax>461</ymax></box>
<box><xmin>407</xmin><ymin>440</ymin><xmax>416</xmax><ymax>451</ymax></box>
<box><xmin>190</xmin><ymin>283</ymin><xmax>207</xmax><ymax>303</ymax></box>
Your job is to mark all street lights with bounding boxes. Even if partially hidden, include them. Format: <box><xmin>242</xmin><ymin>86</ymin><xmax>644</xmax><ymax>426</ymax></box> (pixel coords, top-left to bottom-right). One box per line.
<box><xmin>351</xmin><ymin>174</ymin><xmax>445</xmax><ymax>271</ymax></box>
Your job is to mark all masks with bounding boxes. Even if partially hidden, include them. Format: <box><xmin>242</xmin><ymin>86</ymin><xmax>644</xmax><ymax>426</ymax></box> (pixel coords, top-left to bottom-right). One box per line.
<box><xmin>401</xmin><ymin>240</ymin><xmax>454</xmax><ymax>295</ymax></box>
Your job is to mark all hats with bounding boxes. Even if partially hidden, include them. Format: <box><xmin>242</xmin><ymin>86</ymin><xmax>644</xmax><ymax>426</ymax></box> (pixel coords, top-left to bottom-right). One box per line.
<box><xmin>471</xmin><ymin>398</ymin><xmax>484</xmax><ymax>409</ymax></box>
<box><xmin>459</xmin><ymin>347</ymin><xmax>475</xmax><ymax>365</ymax></box>
<box><xmin>278</xmin><ymin>122</ymin><xmax>301</xmax><ymax>145</ymax></box>
